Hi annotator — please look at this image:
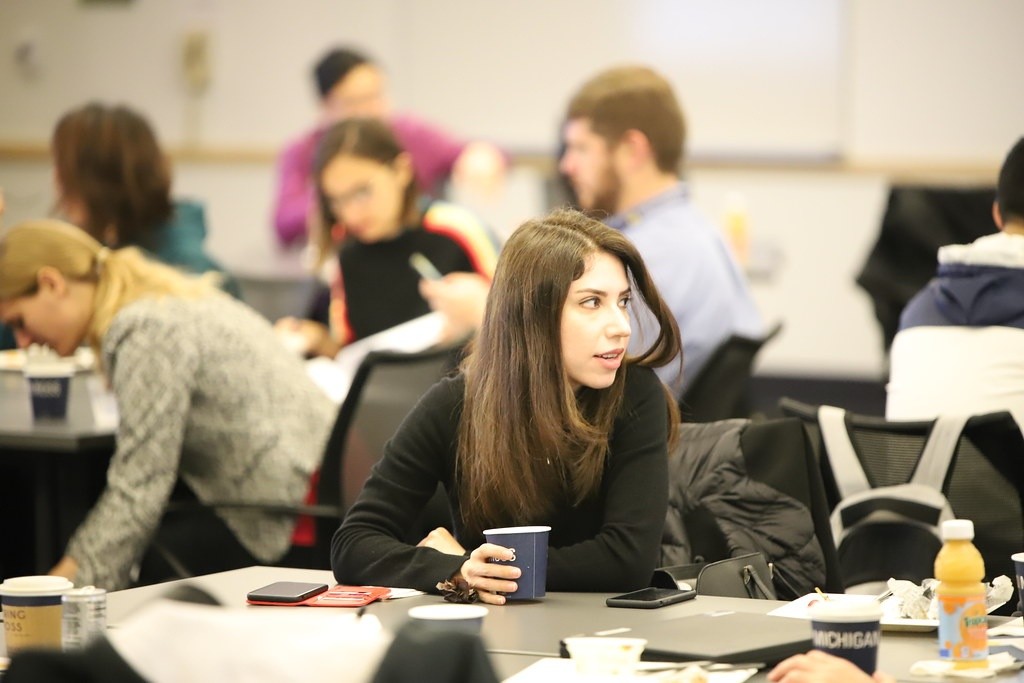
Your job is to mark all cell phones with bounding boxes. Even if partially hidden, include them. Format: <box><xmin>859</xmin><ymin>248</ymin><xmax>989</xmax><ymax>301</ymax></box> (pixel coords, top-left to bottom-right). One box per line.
<box><xmin>248</xmin><ymin>581</ymin><xmax>329</xmax><ymax>603</ymax></box>
<box><xmin>606</xmin><ymin>587</ymin><xmax>695</xmax><ymax>609</ymax></box>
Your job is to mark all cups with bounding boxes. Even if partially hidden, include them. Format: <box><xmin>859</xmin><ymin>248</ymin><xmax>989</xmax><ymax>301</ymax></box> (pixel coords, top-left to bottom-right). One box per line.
<box><xmin>482</xmin><ymin>525</ymin><xmax>553</xmax><ymax>599</ymax></box>
<box><xmin>26</xmin><ymin>364</ymin><xmax>73</xmax><ymax>431</ymax></box>
<box><xmin>1011</xmin><ymin>552</ymin><xmax>1024</xmax><ymax>615</ymax></box>
<box><xmin>60</xmin><ymin>588</ymin><xmax>105</xmax><ymax>654</ymax></box>
<box><xmin>407</xmin><ymin>603</ymin><xmax>489</xmax><ymax>639</ymax></box>
<box><xmin>0</xmin><ymin>575</ymin><xmax>74</xmax><ymax>659</ymax></box>
<box><xmin>808</xmin><ymin>598</ymin><xmax>882</xmax><ymax>678</ymax></box>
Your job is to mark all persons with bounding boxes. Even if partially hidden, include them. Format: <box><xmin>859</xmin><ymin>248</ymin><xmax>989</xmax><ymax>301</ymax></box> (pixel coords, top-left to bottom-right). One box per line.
<box><xmin>274</xmin><ymin>47</ymin><xmax>511</xmax><ymax>251</ymax></box>
<box><xmin>560</xmin><ymin>68</ymin><xmax>763</xmax><ymax>406</ymax></box>
<box><xmin>0</xmin><ymin>217</ymin><xmax>337</xmax><ymax>594</ymax></box>
<box><xmin>330</xmin><ymin>210</ymin><xmax>684</xmax><ymax>605</ymax></box>
<box><xmin>885</xmin><ymin>136</ymin><xmax>1024</xmax><ymax>421</ymax></box>
<box><xmin>0</xmin><ymin>98</ymin><xmax>244</xmax><ymax>349</ymax></box>
<box><xmin>275</xmin><ymin>116</ymin><xmax>494</xmax><ymax>358</ymax></box>
<box><xmin>432</xmin><ymin>137</ymin><xmax>549</xmax><ymax>272</ymax></box>
<box><xmin>766</xmin><ymin>649</ymin><xmax>893</xmax><ymax>683</ymax></box>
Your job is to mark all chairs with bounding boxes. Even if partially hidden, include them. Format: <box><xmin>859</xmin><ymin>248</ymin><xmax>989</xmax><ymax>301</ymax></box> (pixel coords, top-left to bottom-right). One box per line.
<box><xmin>149</xmin><ymin>174</ymin><xmax>1024</xmax><ymax>608</ymax></box>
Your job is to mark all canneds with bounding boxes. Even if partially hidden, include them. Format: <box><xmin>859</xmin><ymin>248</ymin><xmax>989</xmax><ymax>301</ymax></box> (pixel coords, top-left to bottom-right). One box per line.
<box><xmin>60</xmin><ymin>584</ymin><xmax>106</xmax><ymax>652</ymax></box>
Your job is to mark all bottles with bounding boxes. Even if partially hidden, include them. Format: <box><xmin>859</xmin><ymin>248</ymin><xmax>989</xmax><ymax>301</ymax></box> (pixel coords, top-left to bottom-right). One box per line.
<box><xmin>930</xmin><ymin>520</ymin><xmax>989</xmax><ymax>671</ymax></box>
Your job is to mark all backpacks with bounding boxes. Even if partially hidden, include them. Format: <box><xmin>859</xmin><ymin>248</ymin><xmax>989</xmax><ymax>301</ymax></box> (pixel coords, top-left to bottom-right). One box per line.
<box><xmin>817</xmin><ymin>404</ymin><xmax>969</xmax><ymax>596</ymax></box>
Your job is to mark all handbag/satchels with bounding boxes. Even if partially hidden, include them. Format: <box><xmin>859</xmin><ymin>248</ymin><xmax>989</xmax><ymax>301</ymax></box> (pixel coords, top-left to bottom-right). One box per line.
<box><xmin>650</xmin><ymin>551</ymin><xmax>797</xmax><ymax>604</ymax></box>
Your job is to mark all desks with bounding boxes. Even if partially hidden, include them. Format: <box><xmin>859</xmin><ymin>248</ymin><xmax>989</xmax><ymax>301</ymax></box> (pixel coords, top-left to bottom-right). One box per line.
<box><xmin>0</xmin><ymin>336</ymin><xmax>313</xmax><ymax>574</ymax></box>
<box><xmin>0</xmin><ymin>564</ymin><xmax>1024</xmax><ymax>683</ymax></box>
